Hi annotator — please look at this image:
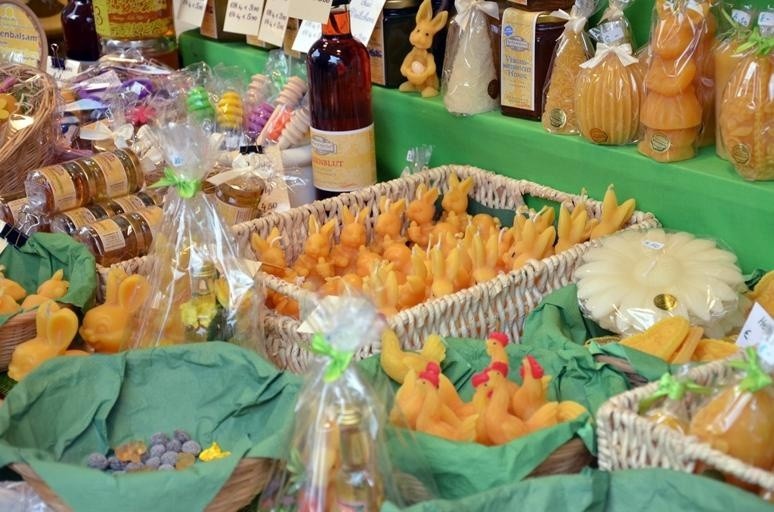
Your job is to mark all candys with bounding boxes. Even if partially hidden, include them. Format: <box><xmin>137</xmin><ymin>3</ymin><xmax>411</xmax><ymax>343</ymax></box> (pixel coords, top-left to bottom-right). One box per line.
<box><xmin>79</xmin><ymin>428</ymin><xmax>201</xmax><ymax>472</ymax></box>
<box><xmin>183</xmin><ymin>72</ymin><xmax>310</xmax><ymax>150</ymax></box>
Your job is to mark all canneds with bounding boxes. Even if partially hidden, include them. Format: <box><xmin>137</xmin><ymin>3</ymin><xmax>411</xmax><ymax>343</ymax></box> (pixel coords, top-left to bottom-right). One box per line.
<box><xmin>0</xmin><ymin>145</ymin><xmax>268</xmax><ymax>267</ymax></box>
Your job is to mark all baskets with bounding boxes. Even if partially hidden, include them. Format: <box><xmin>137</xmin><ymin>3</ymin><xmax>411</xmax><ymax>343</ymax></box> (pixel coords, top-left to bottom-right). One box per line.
<box><xmin>0</xmin><ymin>59</ymin><xmax>58</xmax><ymax>204</ymax></box>
<box><xmin>393</xmin><ymin>434</ymin><xmax>596</xmax><ymax>505</ymax></box>
<box><xmin>597</xmin><ymin>351</ymin><xmax>774</xmax><ymax>501</ymax></box>
<box><xmin>0</xmin><ymin>302</ymin><xmax>75</xmax><ymax>372</ymax></box>
<box><xmin>88</xmin><ymin>252</ymin><xmax>157</xmax><ymax>308</ymax></box>
<box><xmin>210</xmin><ymin>163</ymin><xmax>662</xmax><ymax>372</ymax></box>
<box><xmin>593</xmin><ymin>351</ymin><xmax>650</xmax><ymax>391</ymax></box>
<box><xmin>0</xmin><ymin>56</ymin><xmax>772</xmax><ymax>510</ymax></box>
<box><xmin>10</xmin><ymin>457</ymin><xmax>283</xmax><ymax>512</ymax></box>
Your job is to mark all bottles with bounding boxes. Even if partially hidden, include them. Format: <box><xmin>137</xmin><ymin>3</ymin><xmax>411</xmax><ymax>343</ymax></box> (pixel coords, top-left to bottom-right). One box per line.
<box><xmin>181</xmin><ymin>264</ymin><xmax>231</xmax><ymax>343</ymax></box>
<box><xmin>332</xmin><ymin>402</ymin><xmax>386</xmax><ymax>512</ymax></box>
<box><xmin>60</xmin><ymin>0</ymin><xmax>101</xmax><ymax>66</ymax></box>
<box><xmin>305</xmin><ymin>2</ymin><xmax>380</xmax><ymax>203</ymax></box>
